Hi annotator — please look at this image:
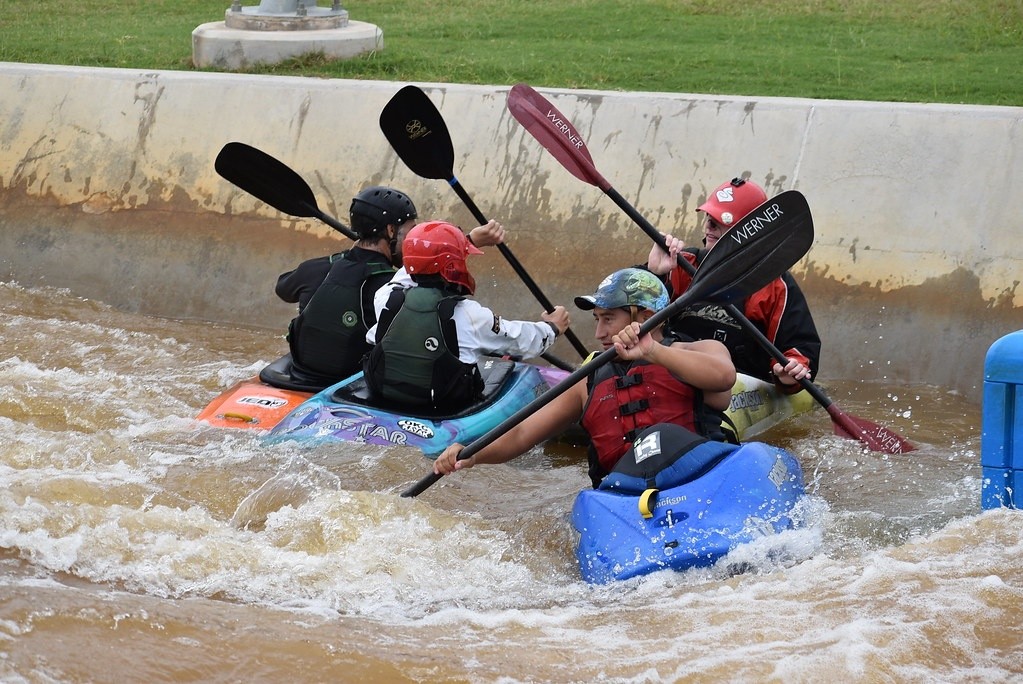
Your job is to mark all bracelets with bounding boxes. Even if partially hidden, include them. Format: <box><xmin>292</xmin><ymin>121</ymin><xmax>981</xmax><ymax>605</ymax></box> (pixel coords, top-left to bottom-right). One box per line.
<box><xmin>465</xmin><ymin>233</ymin><xmax>478</xmax><ymax>248</ymax></box>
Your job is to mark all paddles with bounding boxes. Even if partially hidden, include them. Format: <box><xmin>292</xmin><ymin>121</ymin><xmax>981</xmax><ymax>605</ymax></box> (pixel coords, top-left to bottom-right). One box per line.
<box><xmin>378</xmin><ymin>84</ymin><xmax>591</xmax><ymax>362</ymax></box>
<box><xmin>505</xmin><ymin>81</ymin><xmax>921</xmax><ymax>456</ymax></box>
<box><xmin>214</xmin><ymin>141</ymin><xmax>359</xmax><ymax>244</ymax></box>
<box><xmin>397</xmin><ymin>188</ymin><xmax>816</xmax><ymax>499</ymax></box>
<box><xmin>537</xmin><ymin>346</ymin><xmax>575</xmax><ymax>375</ymax></box>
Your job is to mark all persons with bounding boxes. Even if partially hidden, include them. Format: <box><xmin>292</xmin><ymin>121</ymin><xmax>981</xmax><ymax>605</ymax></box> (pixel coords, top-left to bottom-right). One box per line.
<box><xmin>276</xmin><ymin>187</ymin><xmax>506</xmax><ymax>386</ymax></box>
<box><xmin>362</xmin><ymin>221</ymin><xmax>571</xmax><ymax>417</ymax></box>
<box><xmin>635</xmin><ymin>178</ymin><xmax>823</xmax><ymax>394</ymax></box>
<box><xmin>432</xmin><ymin>267</ymin><xmax>738</xmax><ymax>491</ymax></box>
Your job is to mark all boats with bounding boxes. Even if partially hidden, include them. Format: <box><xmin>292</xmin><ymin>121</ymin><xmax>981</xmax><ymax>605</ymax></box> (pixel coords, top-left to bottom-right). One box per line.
<box><xmin>193</xmin><ymin>349</ymin><xmax>326</xmax><ymax>432</ymax></box>
<box><xmin>566</xmin><ymin>421</ymin><xmax>805</xmax><ymax>597</ymax></box>
<box><xmin>256</xmin><ymin>355</ymin><xmax>574</xmax><ymax>466</ymax></box>
<box><xmin>578</xmin><ymin>351</ymin><xmax>834</xmax><ymax>446</ymax></box>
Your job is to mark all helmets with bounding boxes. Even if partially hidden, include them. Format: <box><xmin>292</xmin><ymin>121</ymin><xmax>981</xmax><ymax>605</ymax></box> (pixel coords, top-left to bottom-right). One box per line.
<box><xmin>402</xmin><ymin>221</ymin><xmax>485</xmax><ymax>296</ymax></box>
<box><xmin>351</xmin><ymin>187</ymin><xmax>419</xmax><ymax>234</ymax></box>
<box><xmin>694</xmin><ymin>177</ymin><xmax>768</xmax><ymax>227</ymax></box>
<box><xmin>574</xmin><ymin>268</ymin><xmax>671</xmax><ymax>326</ymax></box>
